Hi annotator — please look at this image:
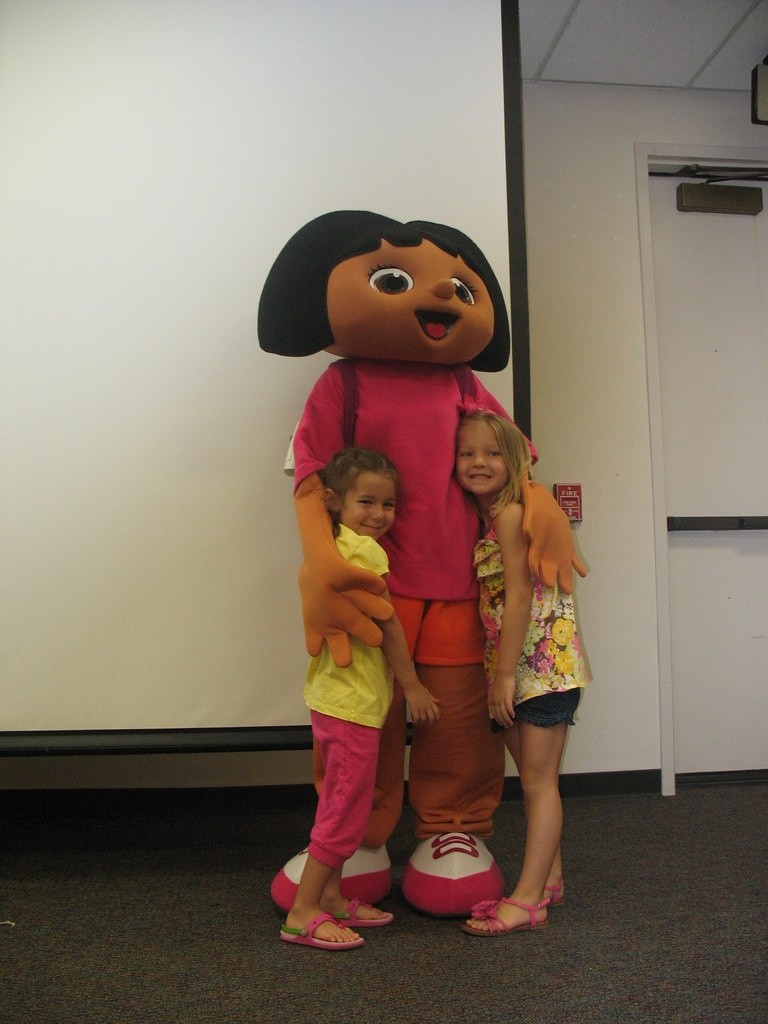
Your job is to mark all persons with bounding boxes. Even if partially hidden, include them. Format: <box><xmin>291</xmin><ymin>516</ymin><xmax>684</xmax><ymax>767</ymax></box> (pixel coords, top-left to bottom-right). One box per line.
<box><xmin>256</xmin><ymin>210</ymin><xmax>587</xmax><ymax>914</ymax></box>
<box><xmin>453</xmin><ymin>409</ymin><xmax>594</xmax><ymax>936</ymax></box>
<box><xmin>280</xmin><ymin>447</ymin><xmax>442</xmax><ymax>950</ymax></box>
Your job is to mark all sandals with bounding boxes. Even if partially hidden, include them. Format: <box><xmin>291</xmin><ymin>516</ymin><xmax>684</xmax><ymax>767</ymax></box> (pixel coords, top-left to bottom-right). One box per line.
<box><xmin>331</xmin><ymin>897</ymin><xmax>394</xmax><ymax>927</ymax></box>
<box><xmin>280</xmin><ymin>912</ymin><xmax>365</xmax><ymax>950</ymax></box>
<box><xmin>543</xmin><ymin>878</ymin><xmax>566</xmax><ymax>907</ymax></box>
<box><xmin>460</xmin><ymin>897</ymin><xmax>551</xmax><ymax>936</ymax></box>
